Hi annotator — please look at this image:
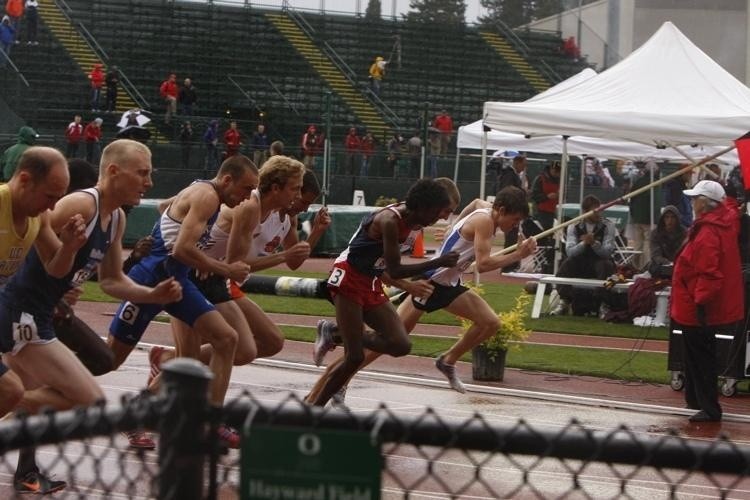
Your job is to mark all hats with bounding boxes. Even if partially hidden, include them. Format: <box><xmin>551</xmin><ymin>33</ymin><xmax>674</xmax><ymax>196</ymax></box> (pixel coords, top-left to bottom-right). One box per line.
<box><xmin>552</xmin><ymin>160</ymin><xmax>561</xmax><ymax>169</ymax></box>
<box><xmin>682</xmin><ymin>180</ymin><xmax>727</xmax><ymax>202</ymax></box>
<box><xmin>582</xmin><ymin>195</ymin><xmax>600</xmax><ymax>212</ymax></box>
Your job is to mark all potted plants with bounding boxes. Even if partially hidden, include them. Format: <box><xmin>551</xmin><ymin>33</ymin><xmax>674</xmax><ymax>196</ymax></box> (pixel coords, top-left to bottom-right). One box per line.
<box><xmin>459</xmin><ymin>282</ymin><xmax>533</xmax><ymax>382</ymax></box>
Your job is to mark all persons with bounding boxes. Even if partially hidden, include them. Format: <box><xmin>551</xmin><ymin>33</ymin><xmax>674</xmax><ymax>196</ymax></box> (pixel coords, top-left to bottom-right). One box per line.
<box><xmin>5</xmin><ymin>0</ymin><xmax>25</xmax><ymax>45</ymax></box>
<box><xmin>564</xmin><ymin>36</ymin><xmax>581</xmax><ymax>64</ymax></box>
<box><xmin>549</xmin><ymin>30</ymin><xmax>568</xmax><ymax>59</ymax></box>
<box><xmin>0</xmin><ymin>14</ymin><xmax>18</xmax><ymax>71</ymax></box>
<box><xmin>23</xmin><ymin>0</ymin><xmax>39</xmax><ymax>47</ymax></box>
<box><xmin>67</xmin><ymin>63</ymin><xmax>238</xmax><ymax>125</ymax></box>
<box><xmin>0</xmin><ymin>110</ymin><xmax>750</xmax><ymax>494</ymax></box>
<box><xmin>367</xmin><ymin>53</ymin><xmax>393</xmax><ymax>106</ymax></box>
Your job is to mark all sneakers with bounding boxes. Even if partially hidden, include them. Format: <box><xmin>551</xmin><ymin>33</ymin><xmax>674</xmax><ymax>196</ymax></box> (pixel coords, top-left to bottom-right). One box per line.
<box><xmin>204</xmin><ymin>424</ymin><xmax>239</xmax><ymax>448</ymax></box>
<box><xmin>645</xmin><ymin>161</ymin><xmax>659</xmax><ymax>172</ymax></box>
<box><xmin>549</xmin><ymin>304</ymin><xmax>568</xmax><ymax>315</ymax></box>
<box><xmin>313</xmin><ymin>319</ymin><xmax>338</xmax><ymax>368</ymax></box>
<box><xmin>14</xmin><ymin>466</ymin><xmax>67</xmax><ymax>496</ymax></box>
<box><xmin>118</xmin><ymin>430</ymin><xmax>155</xmax><ymax>449</ymax></box>
<box><xmin>330</xmin><ymin>385</ymin><xmax>350</xmax><ymax>414</ymax></box>
<box><xmin>434</xmin><ymin>353</ymin><xmax>467</xmax><ymax>394</ymax></box>
<box><xmin>147</xmin><ymin>345</ymin><xmax>169</xmax><ymax>388</ymax></box>
<box><xmin>598</xmin><ymin>302</ymin><xmax>611</xmax><ymax>320</ymax></box>
<box><xmin>688</xmin><ymin>410</ymin><xmax>721</xmax><ymax>422</ymax></box>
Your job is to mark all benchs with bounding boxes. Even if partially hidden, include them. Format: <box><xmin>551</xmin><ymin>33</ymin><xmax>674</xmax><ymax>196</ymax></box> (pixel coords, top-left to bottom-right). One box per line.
<box><xmin>505</xmin><ymin>268</ymin><xmax>673</xmax><ymax>323</ymax></box>
